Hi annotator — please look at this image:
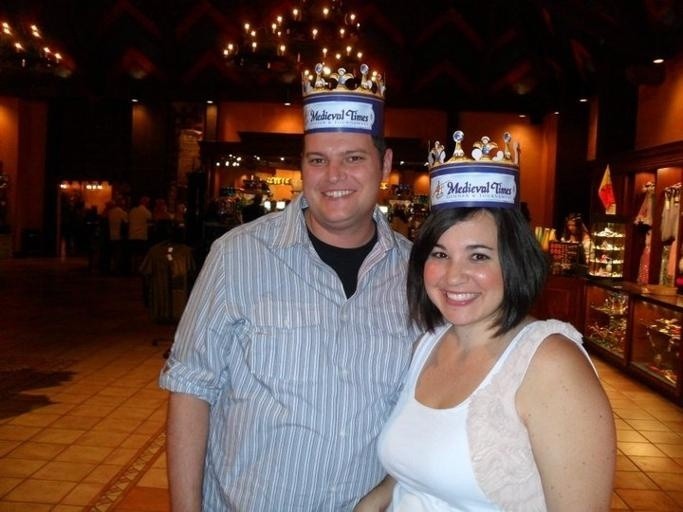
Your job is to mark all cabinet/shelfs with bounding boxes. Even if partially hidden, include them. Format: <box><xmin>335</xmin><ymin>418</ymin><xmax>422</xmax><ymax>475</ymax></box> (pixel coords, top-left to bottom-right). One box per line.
<box><xmin>531</xmin><ymin>142</ymin><xmax>683</xmax><ymax>399</ymax></box>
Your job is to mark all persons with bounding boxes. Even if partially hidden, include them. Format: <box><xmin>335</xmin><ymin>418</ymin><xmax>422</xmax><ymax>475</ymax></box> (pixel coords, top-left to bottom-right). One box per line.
<box><xmin>64</xmin><ymin>193</ymin><xmax>267</xmax><ymax>290</ymax></box>
<box><xmin>158</xmin><ymin>130</ymin><xmax>416</xmax><ymax>510</ymax></box>
<box><xmin>353</xmin><ymin>202</ymin><xmax>615</xmax><ymax>512</ymax></box>
<box><xmin>560</xmin><ymin>213</ymin><xmax>594</xmax><ymax>265</ymax></box>
